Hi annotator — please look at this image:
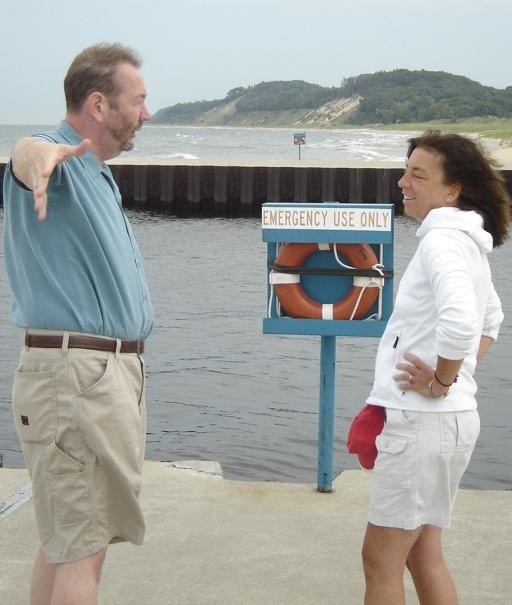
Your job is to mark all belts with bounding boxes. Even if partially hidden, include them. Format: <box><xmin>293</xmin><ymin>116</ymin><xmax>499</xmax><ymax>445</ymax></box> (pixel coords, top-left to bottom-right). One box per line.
<box><xmin>28</xmin><ymin>335</ymin><xmax>144</xmax><ymax>353</ymax></box>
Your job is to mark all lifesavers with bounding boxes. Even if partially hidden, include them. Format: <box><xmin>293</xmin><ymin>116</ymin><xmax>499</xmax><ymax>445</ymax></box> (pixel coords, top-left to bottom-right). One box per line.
<box><xmin>267</xmin><ymin>241</ymin><xmax>382</xmax><ymax>321</ymax></box>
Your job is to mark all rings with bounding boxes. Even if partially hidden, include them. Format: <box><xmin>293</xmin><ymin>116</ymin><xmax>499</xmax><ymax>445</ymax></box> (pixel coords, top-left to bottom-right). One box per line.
<box><xmin>409</xmin><ymin>375</ymin><xmax>412</xmax><ymax>383</ymax></box>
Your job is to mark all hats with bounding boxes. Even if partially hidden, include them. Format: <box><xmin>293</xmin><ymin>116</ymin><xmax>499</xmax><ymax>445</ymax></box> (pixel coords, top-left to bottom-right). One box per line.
<box><xmin>347</xmin><ymin>404</ymin><xmax>384</xmax><ymax>470</ymax></box>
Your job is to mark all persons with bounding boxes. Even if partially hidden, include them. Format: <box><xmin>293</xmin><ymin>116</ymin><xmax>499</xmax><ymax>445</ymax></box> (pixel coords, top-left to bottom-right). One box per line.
<box><xmin>361</xmin><ymin>130</ymin><xmax>511</xmax><ymax>604</ymax></box>
<box><xmin>1</xmin><ymin>43</ymin><xmax>157</xmax><ymax>604</ymax></box>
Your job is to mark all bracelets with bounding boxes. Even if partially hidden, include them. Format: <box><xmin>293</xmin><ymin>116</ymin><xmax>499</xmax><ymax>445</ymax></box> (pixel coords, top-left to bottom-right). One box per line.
<box><xmin>428</xmin><ymin>380</ymin><xmax>449</xmax><ymax>399</ymax></box>
<box><xmin>434</xmin><ymin>372</ymin><xmax>453</xmax><ymax>387</ymax></box>
